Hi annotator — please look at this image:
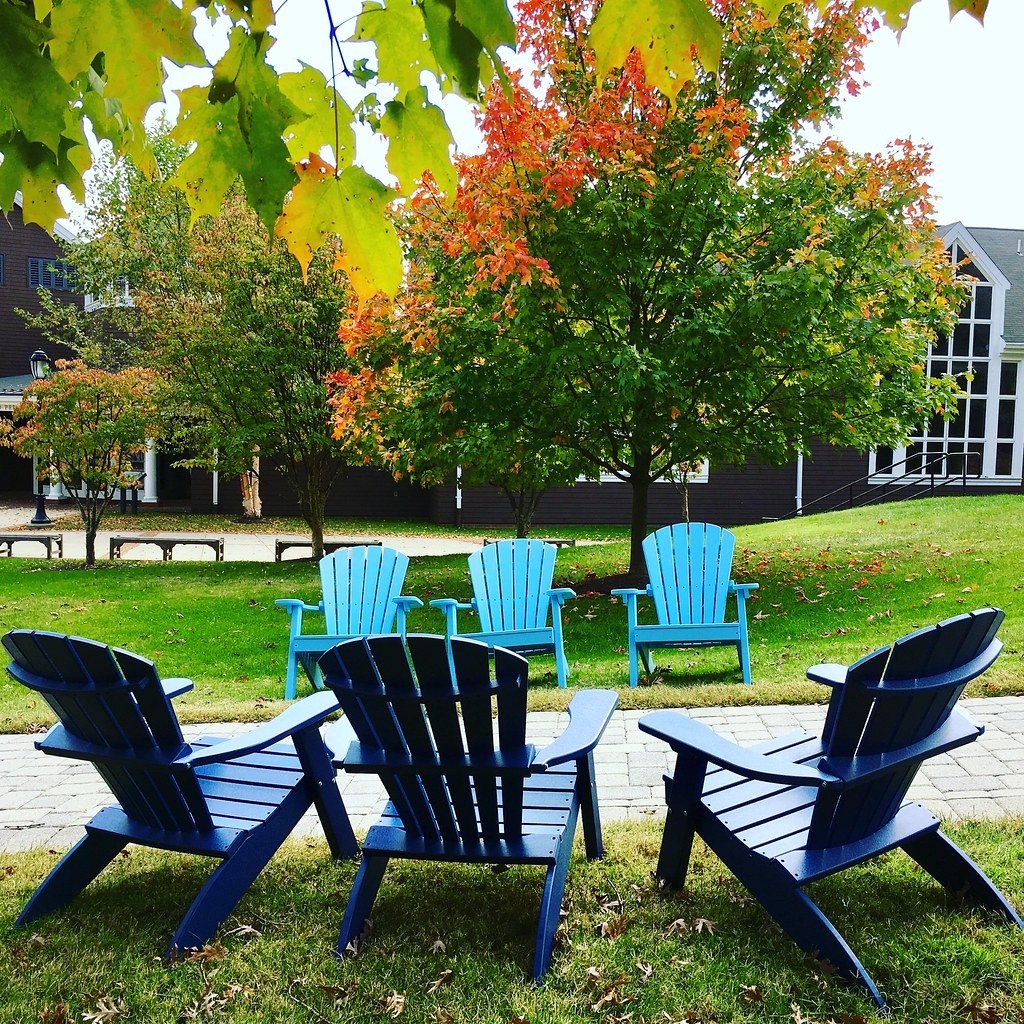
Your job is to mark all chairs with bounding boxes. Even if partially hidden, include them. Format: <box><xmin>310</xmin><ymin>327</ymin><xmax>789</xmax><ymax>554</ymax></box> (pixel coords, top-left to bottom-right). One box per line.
<box><xmin>428</xmin><ymin>539</ymin><xmax>578</xmax><ymax>691</ymax></box>
<box><xmin>315</xmin><ymin>632</ymin><xmax>620</xmax><ymax>981</ymax></box>
<box><xmin>0</xmin><ymin>628</ymin><xmax>360</xmax><ymax>959</ymax></box>
<box><xmin>275</xmin><ymin>546</ymin><xmax>424</xmax><ymax>701</ymax></box>
<box><xmin>611</xmin><ymin>522</ymin><xmax>759</xmax><ymax>689</ymax></box>
<box><xmin>638</xmin><ymin>607</ymin><xmax>1024</xmax><ymax>1004</ymax></box>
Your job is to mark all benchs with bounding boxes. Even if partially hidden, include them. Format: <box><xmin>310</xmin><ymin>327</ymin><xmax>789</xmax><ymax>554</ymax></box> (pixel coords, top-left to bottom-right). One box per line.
<box><xmin>485</xmin><ymin>539</ymin><xmax>575</xmax><ymax>549</ymax></box>
<box><xmin>0</xmin><ymin>533</ymin><xmax>63</xmax><ymax>560</ymax></box>
<box><xmin>110</xmin><ymin>535</ymin><xmax>225</xmax><ymax>562</ymax></box>
<box><xmin>276</xmin><ymin>538</ymin><xmax>382</xmax><ymax>562</ymax></box>
